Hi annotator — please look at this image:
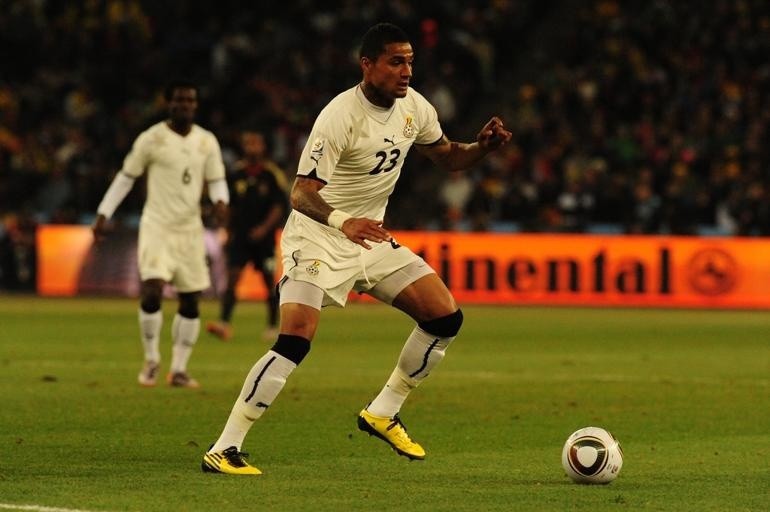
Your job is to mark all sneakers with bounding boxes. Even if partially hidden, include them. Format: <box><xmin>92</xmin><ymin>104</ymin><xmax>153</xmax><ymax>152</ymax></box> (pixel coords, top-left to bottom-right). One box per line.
<box><xmin>202</xmin><ymin>444</ymin><xmax>262</xmax><ymax>474</ymax></box>
<box><xmin>358</xmin><ymin>401</ymin><xmax>426</xmax><ymax>460</ymax></box>
<box><xmin>166</xmin><ymin>370</ymin><xmax>200</xmax><ymax>389</ymax></box>
<box><xmin>139</xmin><ymin>360</ymin><xmax>160</xmax><ymax>386</ymax></box>
<box><xmin>206</xmin><ymin>320</ymin><xmax>232</xmax><ymax>341</ymax></box>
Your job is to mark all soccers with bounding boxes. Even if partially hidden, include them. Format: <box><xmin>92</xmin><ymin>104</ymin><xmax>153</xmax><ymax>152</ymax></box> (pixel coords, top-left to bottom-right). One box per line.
<box><xmin>562</xmin><ymin>427</ymin><xmax>624</xmax><ymax>485</ymax></box>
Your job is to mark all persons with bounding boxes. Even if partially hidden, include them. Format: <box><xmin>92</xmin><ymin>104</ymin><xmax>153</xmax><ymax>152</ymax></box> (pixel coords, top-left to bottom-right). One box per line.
<box><xmin>206</xmin><ymin>132</ymin><xmax>294</xmax><ymax>341</ymax></box>
<box><xmin>201</xmin><ymin>23</ymin><xmax>513</xmax><ymax>475</ymax></box>
<box><xmin>92</xmin><ymin>82</ymin><xmax>230</xmax><ymax>389</ymax></box>
<box><xmin>1</xmin><ymin>1</ymin><xmax>769</xmax><ymax>290</ymax></box>
